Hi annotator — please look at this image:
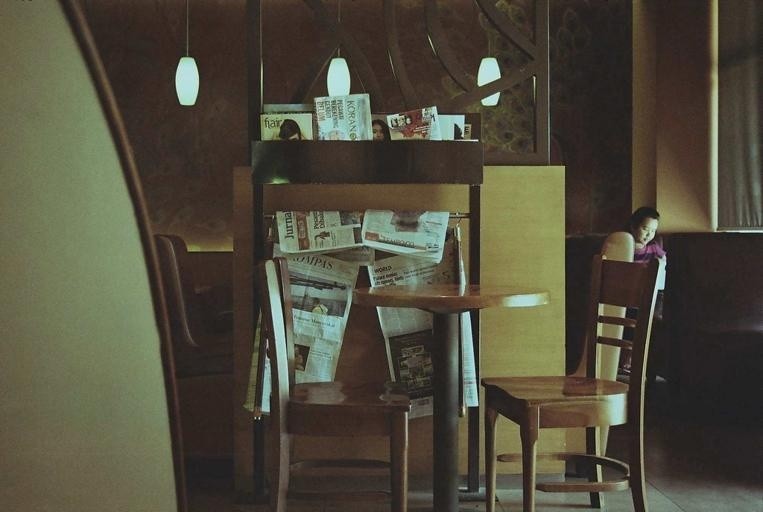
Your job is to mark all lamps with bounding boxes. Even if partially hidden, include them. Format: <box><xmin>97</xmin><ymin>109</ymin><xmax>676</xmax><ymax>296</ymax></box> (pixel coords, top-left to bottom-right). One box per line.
<box><xmin>174</xmin><ymin>0</ymin><xmax>199</xmax><ymax>107</ymax></box>
<box><xmin>476</xmin><ymin>1</ymin><xmax>502</xmax><ymax>107</ymax></box>
<box><xmin>326</xmin><ymin>0</ymin><xmax>351</xmax><ymax>97</ymax></box>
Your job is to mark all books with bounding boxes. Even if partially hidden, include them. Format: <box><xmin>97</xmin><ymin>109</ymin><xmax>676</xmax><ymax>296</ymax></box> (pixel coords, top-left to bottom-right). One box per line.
<box><xmin>386</xmin><ymin>106</ymin><xmax>442</xmax><ymax>142</ymax></box>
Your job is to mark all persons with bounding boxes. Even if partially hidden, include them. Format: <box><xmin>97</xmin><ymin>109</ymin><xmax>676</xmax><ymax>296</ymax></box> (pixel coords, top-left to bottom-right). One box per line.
<box><xmin>275</xmin><ymin>118</ymin><xmax>303</xmax><ymax>142</ymax></box>
<box><xmin>310</xmin><ymin>296</ymin><xmax>329</xmax><ymax>316</ymax></box>
<box><xmin>616</xmin><ymin>205</ymin><xmax>667</xmax><ymax>371</ymax></box>
<box><xmin>294</xmin><ymin>346</ymin><xmax>305</xmax><ymax>371</ymax></box>
<box><xmin>368</xmin><ymin>118</ymin><xmax>391</xmax><ymax>142</ymax></box>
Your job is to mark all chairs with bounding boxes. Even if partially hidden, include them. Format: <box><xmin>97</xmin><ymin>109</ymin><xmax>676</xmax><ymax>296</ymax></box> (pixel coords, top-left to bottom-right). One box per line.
<box><xmin>153</xmin><ymin>234</ymin><xmax>237</xmax><ymax>461</ymax></box>
<box><xmin>257</xmin><ymin>254</ymin><xmax>412</xmax><ymax>511</ymax></box>
<box><xmin>478</xmin><ymin>253</ymin><xmax>659</xmax><ymax>511</ymax></box>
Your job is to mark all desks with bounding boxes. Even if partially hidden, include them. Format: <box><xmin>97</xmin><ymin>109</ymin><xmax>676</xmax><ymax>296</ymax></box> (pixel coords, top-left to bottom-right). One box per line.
<box><xmin>349</xmin><ymin>284</ymin><xmax>552</xmax><ymax>511</ymax></box>
